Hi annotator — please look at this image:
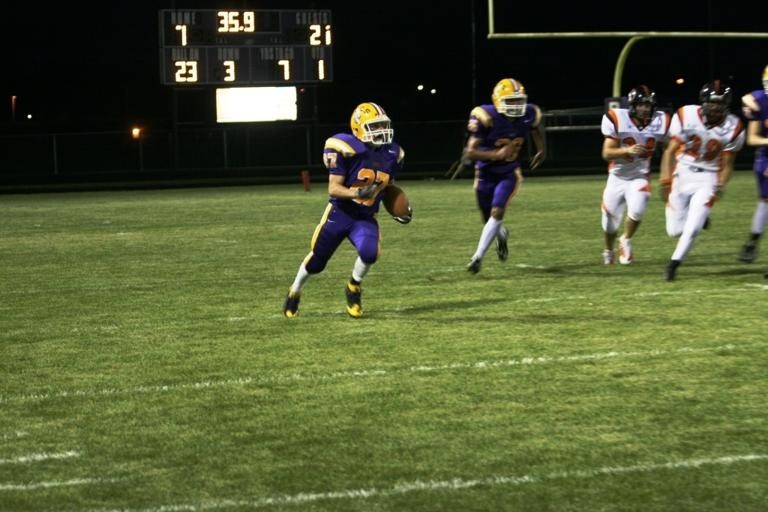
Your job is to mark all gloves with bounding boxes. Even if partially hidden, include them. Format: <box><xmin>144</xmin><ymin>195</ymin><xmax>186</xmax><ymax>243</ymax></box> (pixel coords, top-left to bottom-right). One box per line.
<box><xmin>656</xmin><ymin>177</ymin><xmax>675</xmax><ymax>204</ymax></box>
<box><xmin>392</xmin><ymin>206</ymin><xmax>414</xmax><ymax>223</ymax></box>
<box><xmin>358</xmin><ymin>181</ymin><xmax>378</xmax><ymax>199</ymax></box>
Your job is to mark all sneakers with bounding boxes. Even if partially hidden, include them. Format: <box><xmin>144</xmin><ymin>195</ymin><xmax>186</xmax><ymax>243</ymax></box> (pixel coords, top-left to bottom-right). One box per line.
<box><xmin>342</xmin><ymin>278</ymin><xmax>366</xmax><ymax>319</ymax></box>
<box><xmin>494</xmin><ymin>225</ymin><xmax>510</xmax><ymax>264</ymax></box>
<box><xmin>465</xmin><ymin>256</ymin><xmax>482</xmax><ymax>275</ymax></box>
<box><xmin>603</xmin><ymin>246</ymin><xmax>615</xmax><ymax>266</ymax></box>
<box><xmin>737</xmin><ymin>236</ymin><xmax>760</xmax><ymax>263</ymax></box>
<box><xmin>664</xmin><ymin>258</ymin><xmax>683</xmax><ymax>282</ymax></box>
<box><xmin>280</xmin><ymin>282</ymin><xmax>302</xmax><ymax>319</ymax></box>
<box><xmin>618</xmin><ymin>234</ymin><xmax>634</xmax><ymax>265</ymax></box>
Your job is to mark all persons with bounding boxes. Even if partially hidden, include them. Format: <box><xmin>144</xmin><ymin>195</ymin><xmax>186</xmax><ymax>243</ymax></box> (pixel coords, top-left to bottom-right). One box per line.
<box><xmin>596</xmin><ymin>86</ymin><xmax>671</xmax><ymax>266</ymax></box>
<box><xmin>282</xmin><ymin>99</ymin><xmax>406</xmax><ymax>319</ymax></box>
<box><xmin>657</xmin><ymin>79</ymin><xmax>745</xmax><ymax>284</ymax></box>
<box><xmin>461</xmin><ymin>78</ymin><xmax>547</xmax><ymax>275</ymax></box>
<box><xmin>733</xmin><ymin>63</ymin><xmax>767</xmax><ymax>263</ymax></box>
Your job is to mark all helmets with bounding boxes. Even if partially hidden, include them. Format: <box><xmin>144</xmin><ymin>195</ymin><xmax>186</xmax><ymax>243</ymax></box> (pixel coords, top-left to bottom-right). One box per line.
<box><xmin>627</xmin><ymin>83</ymin><xmax>656</xmax><ymax>122</ymax></box>
<box><xmin>349</xmin><ymin>100</ymin><xmax>397</xmax><ymax>147</ymax></box>
<box><xmin>491</xmin><ymin>76</ymin><xmax>530</xmax><ymax>120</ymax></box>
<box><xmin>699</xmin><ymin>79</ymin><xmax>733</xmax><ymax>123</ymax></box>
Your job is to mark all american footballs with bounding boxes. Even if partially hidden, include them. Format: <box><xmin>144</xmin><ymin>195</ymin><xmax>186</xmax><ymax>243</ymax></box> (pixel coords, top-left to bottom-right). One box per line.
<box><xmin>382</xmin><ymin>186</ymin><xmax>409</xmax><ymax>218</ymax></box>
